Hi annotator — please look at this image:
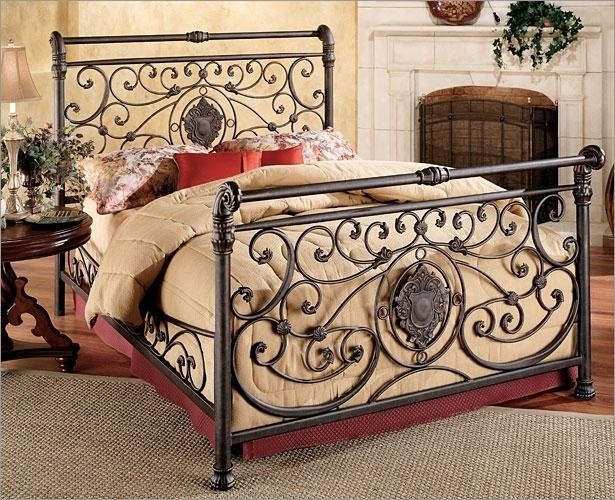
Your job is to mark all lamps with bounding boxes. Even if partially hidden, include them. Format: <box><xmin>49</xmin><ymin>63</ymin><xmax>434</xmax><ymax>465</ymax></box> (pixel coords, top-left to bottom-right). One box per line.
<box><xmin>0</xmin><ymin>38</ymin><xmax>43</xmax><ymax>223</ymax></box>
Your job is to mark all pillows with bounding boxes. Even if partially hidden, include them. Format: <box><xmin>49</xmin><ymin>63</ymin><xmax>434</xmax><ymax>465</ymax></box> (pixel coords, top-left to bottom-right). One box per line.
<box><xmin>83</xmin><ymin>127</ymin><xmax>362</xmax><ymax>216</ymax></box>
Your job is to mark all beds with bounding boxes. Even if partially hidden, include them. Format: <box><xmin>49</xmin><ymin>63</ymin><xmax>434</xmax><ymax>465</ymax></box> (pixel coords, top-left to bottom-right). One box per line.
<box><xmin>50</xmin><ymin>25</ymin><xmax>606</xmax><ymax>492</ymax></box>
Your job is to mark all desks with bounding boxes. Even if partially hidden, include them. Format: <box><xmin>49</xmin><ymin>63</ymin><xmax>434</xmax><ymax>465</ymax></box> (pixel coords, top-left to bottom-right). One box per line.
<box><xmin>0</xmin><ymin>205</ymin><xmax>93</xmax><ymax>372</ymax></box>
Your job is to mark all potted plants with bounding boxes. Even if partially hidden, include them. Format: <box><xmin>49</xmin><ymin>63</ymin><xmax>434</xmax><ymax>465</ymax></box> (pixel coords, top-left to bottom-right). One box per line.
<box><xmin>0</xmin><ymin>114</ymin><xmax>96</xmax><ymax>212</ymax></box>
<box><xmin>492</xmin><ymin>0</ymin><xmax>584</xmax><ymax>71</ymax></box>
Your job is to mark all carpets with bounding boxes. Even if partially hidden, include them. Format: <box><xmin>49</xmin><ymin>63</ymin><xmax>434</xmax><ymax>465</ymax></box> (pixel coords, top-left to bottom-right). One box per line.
<box><xmin>0</xmin><ymin>368</ymin><xmax>615</xmax><ymax>500</ymax></box>
